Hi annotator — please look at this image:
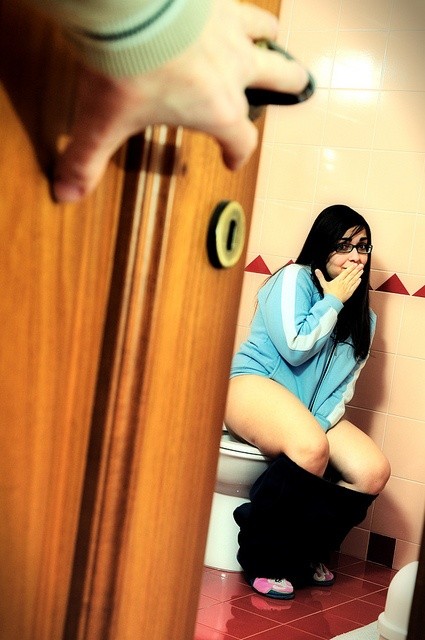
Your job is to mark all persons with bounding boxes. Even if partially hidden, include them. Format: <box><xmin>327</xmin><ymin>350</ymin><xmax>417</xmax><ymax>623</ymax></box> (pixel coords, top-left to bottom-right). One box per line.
<box><xmin>220</xmin><ymin>203</ymin><xmax>394</xmax><ymax>601</ymax></box>
<box><xmin>20</xmin><ymin>2</ymin><xmax>311</xmax><ymax>206</ymax></box>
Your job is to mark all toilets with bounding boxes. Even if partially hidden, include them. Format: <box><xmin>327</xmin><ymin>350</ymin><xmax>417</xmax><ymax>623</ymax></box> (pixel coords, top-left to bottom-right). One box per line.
<box><xmin>203</xmin><ymin>433</ymin><xmax>271</xmax><ymax>573</ymax></box>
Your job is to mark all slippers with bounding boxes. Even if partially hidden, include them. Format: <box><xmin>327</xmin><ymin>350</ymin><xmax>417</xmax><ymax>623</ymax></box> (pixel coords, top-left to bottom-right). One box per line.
<box><xmin>250</xmin><ymin>577</ymin><xmax>294</xmax><ymax>600</ymax></box>
<box><xmin>314</xmin><ymin>563</ymin><xmax>335</xmax><ymax>586</ymax></box>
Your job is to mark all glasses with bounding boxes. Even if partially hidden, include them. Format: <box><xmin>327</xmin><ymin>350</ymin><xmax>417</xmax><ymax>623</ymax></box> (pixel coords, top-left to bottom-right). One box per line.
<box><xmin>336</xmin><ymin>242</ymin><xmax>372</xmax><ymax>254</ymax></box>
